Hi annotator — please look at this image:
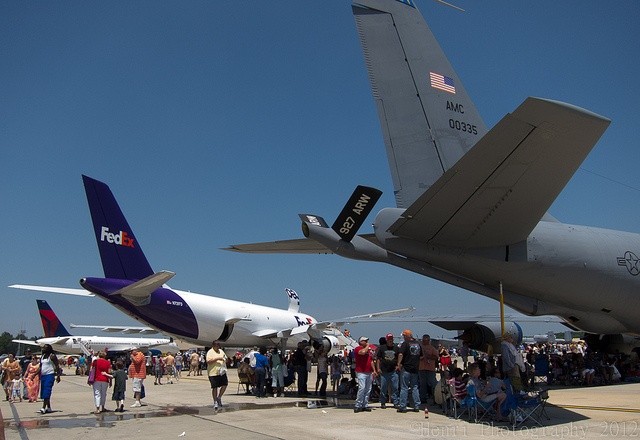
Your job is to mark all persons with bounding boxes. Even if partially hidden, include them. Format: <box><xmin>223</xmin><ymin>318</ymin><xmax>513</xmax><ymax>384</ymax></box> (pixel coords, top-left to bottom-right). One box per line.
<box><xmin>351</xmin><ymin>348</ymin><xmax>355</xmax><ymax>359</ymax></box>
<box><xmin>237</xmin><ymin>339</ymin><xmax>315</xmax><ymax>398</ymax></box>
<box><xmin>348</xmin><ymin>349</ymin><xmax>351</xmax><ymax>357</ymax></box>
<box><xmin>500</xmin><ymin>333</ymin><xmax>525</xmax><ymax>390</ymax></box>
<box><xmin>376</xmin><ymin>332</ymin><xmax>399</xmax><ymax>409</ymax></box>
<box><xmin>0</xmin><ymin>352</ymin><xmax>22</xmax><ymax>402</ymax></box>
<box><xmin>205</xmin><ymin>339</ymin><xmax>229</xmax><ymax>410</ymax></box>
<box><xmin>343</xmin><ymin>348</ymin><xmax>348</xmax><ymax>362</ymax></box>
<box><xmin>318</xmin><ymin>353</ymin><xmax>328</xmax><ymax>396</ymax></box>
<box><xmin>379</xmin><ymin>336</ymin><xmax>386</xmax><ymax>345</ymax></box>
<box><xmin>419</xmin><ymin>334</ymin><xmax>439</xmax><ymax>406</ymax></box>
<box><xmin>29</xmin><ymin>344</ymin><xmax>61</xmax><ymax>414</ymax></box>
<box><xmin>436</xmin><ymin>342</ymin><xmax>500</xmax><ymax>370</ymax></box>
<box><xmin>19</xmin><ymin>348</ymin><xmax>33</xmax><ymax>376</ymax></box>
<box><xmin>10</xmin><ymin>372</ymin><xmax>23</xmax><ymax>403</ymax></box>
<box><xmin>113</xmin><ymin>353</ymin><xmax>132</xmax><ymax>367</ymax></box>
<box><xmin>464</xmin><ymin>373</ymin><xmax>469</xmax><ymax>381</ymax></box>
<box><xmin>148</xmin><ymin>349</ymin><xmax>205</xmax><ymax>384</ymax></box>
<box><xmin>87</xmin><ymin>351</ymin><xmax>112</xmax><ymax>414</ymax></box>
<box><xmin>448</xmin><ymin>367</ymin><xmax>467</xmax><ymax>401</ymax></box>
<box><xmin>63</xmin><ymin>349</ymin><xmax>99</xmax><ymax>376</ymax></box>
<box><xmin>23</xmin><ymin>356</ymin><xmax>41</xmax><ymax>404</ymax></box>
<box><xmin>127</xmin><ymin>346</ymin><xmax>147</xmax><ymax>408</ymax></box>
<box><xmin>233</xmin><ymin>355</ymin><xmax>237</xmax><ymax>366</ymax></box>
<box><xmin>101</xmin><ymin>360</ymin><xmax>128</xmax><ymax>412</ymax></box>
<box><xmin>339</xmin><ymin>355</ymin><xmax>342</xmax><ymax>360</ymax></box>
<box><xmin>522</xmin><ymin>339</ymin><xmax>640</xmax><ymax>390</ymax></box>
<box><xmin>466</xmin><ymin>363</ymin><xmax>507</xmax><ymax>421</ymax></box>
<box><xmin>314</xmin><ymin>349</ymin><xmax>329</xmax><ymax>393</ymax></box>
<box><xmin>395</xmin><ymin>329</ymin><xmax>422</xmax><ymax>413</ymax></box>
<box><xmin>353</xmin><ymin>336</ymin><xmax>376</xmax><ymax>412</ymax></box>
<box><xmin>330</xmin><ymin>355</ymin><xmax>346</xmax><ymax>396</ymax></box>
<box><xmin>332</xmin><ymin>353</ymin><xmax>335</xmax><ymax>358</ymax></box>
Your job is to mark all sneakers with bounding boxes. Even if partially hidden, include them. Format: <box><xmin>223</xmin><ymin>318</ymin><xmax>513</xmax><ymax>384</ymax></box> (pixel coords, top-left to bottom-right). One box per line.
<box><xmin>394</xmin><ymin>404</ymin><xmax>400</xmax><ymax>408</ymax></box>
<box><xmin>397</xmin><ymin>409</ymin><xmax>406</xmax><ymax>413</ymax></box>
<box><xmin>130</xmin><ymin>402</ymin><xmax>141</xmax><ymax>407</ymax></box>
<box><xmin>304</xmin><ymin>391</ymin><xmax>312</xmax><ymax>395</ymax></box>
<box><xmin>298</xmin><ymin>393</ymin><xmax>307</xmax><ymax>397</ymax></box>
<box><xmin>115</xmin><ymin>408</ymin><xmax>119</xmax><ymax>412</ymax></box>
<box><xmin>46</xmin><ymin>408</ymin><xmax>51</xmax><ymax>413</ymax></box>
<box><xmin>274</xmin><ymin>394</ymin><xmax>277</xmax><ymax>397</ymax></box>
<box><xmin>40</xmin><ymin>408</ymin><xmax>45</xmax><ymax>414</ymax></box>
<box><xmin>94</xmin><ymin>409</ymin><xmax>100</xmax><ymax>414</ymax></box>
<box><xmin>217</xmin><ymin>398</ymin><xmax>222</xmax><ymax>405</ymax></box>
<box><xmin>102</xmin><ymin>408</ymin><xmax>106</xmax><ymax>412</ymax></box>
<box><xmin>120</xmin><ymin>403</ymin><xmax>124</xmax><ymax>411</ymax></box>
<box><xmin>362</xmin><ymin>408</ymin><xmax>371</xmax><ymax>411</ymax></box>
<box><xmin>280</xmin><ymin>393</ymin><xmax>285</xmax><ymax>397</ymax></box>
<box><xmin>354</xmin><ymin>407</ymin><xmax>359</xmax><ymax>412</ymax></box>
<box><xmin>214</xmin><ymin>403</ymin><xmax>218</xmax><ymax>409</ymax></box>
<box><xmin>380</xmin><ymin>403</ymin><xmax>385</xmax><ymax>409</ymax></box>
<box><xmin>414</xmin><ymin>408</ymin><xmax>419</xmax><ymax>411</ymax></box>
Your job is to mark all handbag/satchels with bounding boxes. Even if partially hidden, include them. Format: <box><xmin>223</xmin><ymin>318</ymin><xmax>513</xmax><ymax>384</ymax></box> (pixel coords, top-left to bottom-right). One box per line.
<box><xmin>134</xmin><ymin>384</ymin><xmax>145</xmax><ymax>398</ymax></box>
<box><xmin>87</xmin><ymin>366</ymin><xmax>96</xmax><ymax>384</ymax></box>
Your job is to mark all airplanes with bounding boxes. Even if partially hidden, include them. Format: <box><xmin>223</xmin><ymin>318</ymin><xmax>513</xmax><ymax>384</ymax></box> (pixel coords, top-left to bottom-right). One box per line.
<box><xmin>12</xmin><ymin>300</ymin><xmax>207</xmax><ymax>358</ymax></box>
<box><xmin>220</xmin><ymin>1</ymin><xmax>639</xmax><ymax>378</ymax></box>
<box><xmin>9</xmin><ymin>175</ymin><xmax>360</xmax><ymax>357</ymax></box>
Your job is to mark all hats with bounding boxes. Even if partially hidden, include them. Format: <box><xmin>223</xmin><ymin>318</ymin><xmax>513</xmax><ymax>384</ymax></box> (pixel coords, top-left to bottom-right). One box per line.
<box><xmin>403</xmin><ymin>330</ymin><xmax>411</xmax><ymax>335</ymax></box>
<box><xmin>359</xmin><ymin>336</ymin><xmax>369</xmax><ymax>341</ymax></box>
<box><xmin>177</xmin><ymin>351</ymin><xmax>182</xmax><ymax>355</ymax></box>
<box><xmin>386</xmin><ymin>333</ymin><xmax>393</xmax><ymax>340</ymax></box>
<box><xmin>244</xmin><ymin>357</ymin><xmax>250</xmax><ymax>362</ymax></box>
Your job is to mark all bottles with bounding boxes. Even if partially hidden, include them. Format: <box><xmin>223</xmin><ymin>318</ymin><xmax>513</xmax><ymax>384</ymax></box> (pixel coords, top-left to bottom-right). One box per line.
<box><xmin>425</xmin><ymin>407</ymin><xmax>428</xmax><ymax>419</ymax></box>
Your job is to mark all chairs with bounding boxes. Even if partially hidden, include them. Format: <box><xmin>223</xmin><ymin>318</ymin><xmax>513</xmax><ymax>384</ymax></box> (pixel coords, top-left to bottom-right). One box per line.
<box><xmin>467</xmin><ymin>384</ymin><xmax>500</xmax><ymax>425</ymax></box>
<box><xmin>283</xmin><ymin>376</ymin><xmax>295</xmax><ymax>395</ymax></box>
<box><xmin>445</xmin><ymin>390</ymin><xmax>468</xmax><ymax>419</ymax></box>
<box><xmin>533</xmin><ymin>354</ymin><xmax>551</xmax><ymax>386</ymax></box>
<box><xmin>504</xmin><ymin>380</ymin><xmax>544</xmax><ymax>427</ymax></box>
<box><xmin>238</xmin><ymin>371</ymin><xmax>256</xmax><ymax>394</ymax></box>
